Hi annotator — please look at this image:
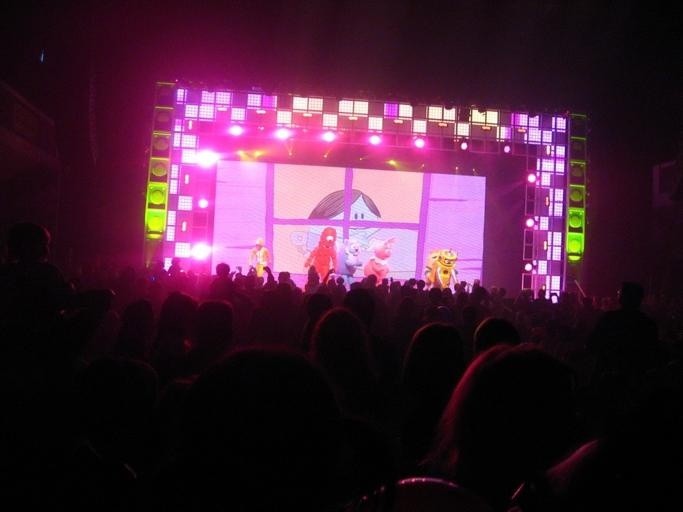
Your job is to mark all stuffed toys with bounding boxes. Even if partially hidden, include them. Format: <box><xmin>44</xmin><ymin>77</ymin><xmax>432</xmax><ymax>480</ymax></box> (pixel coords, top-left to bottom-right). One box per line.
<box><xmin>364</xmin><ymin>237</ymin><xmax>395</xmax><ymax>285</ymax></box>
<box><xmin>425</xmin><ymin>249</ymin><xmax>458</xmax><ymax>289</ymax></box>
<box><xmin>305</xmin><ymin>227</ymin><xmax>338</xmax><ymax>282</ymax></box>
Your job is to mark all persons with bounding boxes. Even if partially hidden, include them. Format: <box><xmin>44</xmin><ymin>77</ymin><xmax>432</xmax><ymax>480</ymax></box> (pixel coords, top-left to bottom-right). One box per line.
<box><xmin>249</xmin><ymin>238</ymin><xmax>271</xmax><ymax>277</ymax></box>
